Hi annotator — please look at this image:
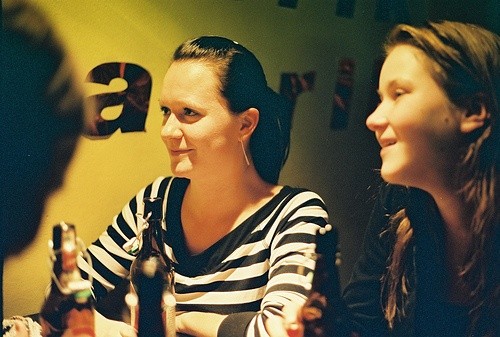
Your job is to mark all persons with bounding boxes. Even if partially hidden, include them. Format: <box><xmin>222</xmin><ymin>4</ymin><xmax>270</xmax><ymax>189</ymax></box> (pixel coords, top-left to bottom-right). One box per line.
<box><xmin>39</xmin><ymin>35</ymin><xmax>330</xmax><ymax>337</ymax></box>
<box><xmin>264</xmin><ymin>19</ymin><xmax>500</xmax><ymax>337</ymax></box>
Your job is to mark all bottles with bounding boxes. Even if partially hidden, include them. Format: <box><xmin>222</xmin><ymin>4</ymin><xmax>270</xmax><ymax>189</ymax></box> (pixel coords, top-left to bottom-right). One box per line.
<box><xmin>130</xmin><ymin>197</ymin><xmax>176</xmax><ymax>337</ymax></box>
<box><xmin>39</xmin><ymin>223</ymin><xmax>95</xmax><ymax>337</ymax></box>
